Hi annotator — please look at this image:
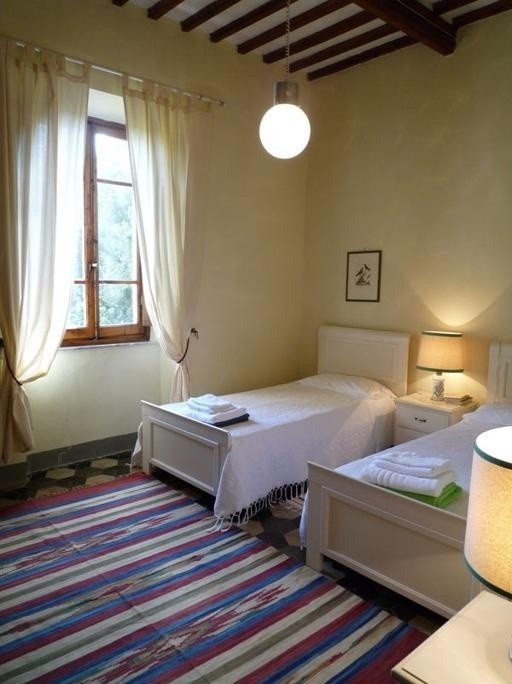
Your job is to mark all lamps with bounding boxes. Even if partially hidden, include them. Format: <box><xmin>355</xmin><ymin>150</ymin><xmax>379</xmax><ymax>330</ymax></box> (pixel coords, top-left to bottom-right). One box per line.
<box><xmin>415</xmin><ymin>329</ymin><xmax>464</xmax><ymax>401</ymax></box>
<box><xmin>259</xmin><ymin>0</ymin><xmax>312</xmax><ymax>162</ymax></box>
<box><xmin>462</xmin><ymin>428</ymin><xmax>510</xmax><ymax>660</ymax></box>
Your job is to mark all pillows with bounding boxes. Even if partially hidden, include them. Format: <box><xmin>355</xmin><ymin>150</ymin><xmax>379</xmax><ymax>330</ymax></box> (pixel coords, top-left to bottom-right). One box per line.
<box><xmin>463</xmin><ymin>403</ymin><xmax>512</xmax><ymax>425</ymax></box>
<box><xmin>299</xmin><ymin>372</ymin><xmax>398</xmax><ymax>399</ymax></box>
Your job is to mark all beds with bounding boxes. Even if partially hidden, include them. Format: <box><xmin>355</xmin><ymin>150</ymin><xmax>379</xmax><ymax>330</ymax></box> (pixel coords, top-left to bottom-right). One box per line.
<box><xmin>301</xmin><ymin>340</ymin><xmax>510</xmax><ymax>621</ymax></box>
<box><xmin>138</xmin><ymin>321</ymin><xmax>412</xmax><ymax>533</ymax></box>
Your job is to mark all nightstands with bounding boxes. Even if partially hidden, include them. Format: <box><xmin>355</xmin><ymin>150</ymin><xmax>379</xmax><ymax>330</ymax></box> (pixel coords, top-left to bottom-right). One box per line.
<box><xmin>394</xmin><ymin>392</ymin><xmax>479</xmax><ymax>445</ymax></box>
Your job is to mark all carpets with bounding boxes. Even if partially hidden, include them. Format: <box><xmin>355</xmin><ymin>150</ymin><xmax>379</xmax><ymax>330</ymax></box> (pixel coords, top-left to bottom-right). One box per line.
<box><xmin>0</xmin><ymin>470</ymin><xmax>409</xmax><ymax>684</ymax></box>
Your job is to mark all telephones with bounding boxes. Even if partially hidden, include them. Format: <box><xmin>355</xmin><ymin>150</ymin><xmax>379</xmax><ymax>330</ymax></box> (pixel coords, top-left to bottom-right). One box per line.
<box><xmin>446</xmin><ymin>394</ymin><xmax>473</xmax><ymax>405</ymax></box>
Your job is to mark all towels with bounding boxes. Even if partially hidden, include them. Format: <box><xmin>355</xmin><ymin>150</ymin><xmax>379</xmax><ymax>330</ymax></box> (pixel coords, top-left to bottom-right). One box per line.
<box><xmin>359</xmin><ymin>451</ymin><xmax>463</xmax><ymax>509</ymax></box>
<box><xmin>179</xmin><ymin>393</ymin><xmax>251</xmax><ymax>428</ymax></box>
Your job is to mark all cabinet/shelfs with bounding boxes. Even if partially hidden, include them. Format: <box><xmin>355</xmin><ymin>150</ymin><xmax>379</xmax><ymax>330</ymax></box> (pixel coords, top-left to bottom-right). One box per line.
<box><xmin>392</xmin><ymin>588</ymin><xmax>512</xmax><ymax>684</ymax></box>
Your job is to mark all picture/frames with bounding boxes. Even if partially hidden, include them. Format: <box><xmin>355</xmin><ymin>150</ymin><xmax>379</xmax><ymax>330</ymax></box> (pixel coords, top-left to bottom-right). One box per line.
<box><xmin>346</xmin><ymin>249</ymin><xmax>382</xmax><ymax>302</ymax></box>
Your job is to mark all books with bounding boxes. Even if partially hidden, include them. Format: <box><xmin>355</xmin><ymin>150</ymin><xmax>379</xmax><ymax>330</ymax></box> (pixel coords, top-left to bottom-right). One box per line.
<box><xmin>444</xmin><ymin>393</ymin><xmax>469</xmax><ymax>400</ymax></box>
<box><xmin>445</xmin><ymin>396</ymin><xmax>472</xmax><ymax>405</ymax></box>
